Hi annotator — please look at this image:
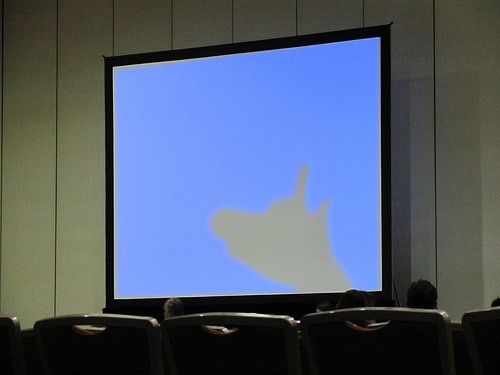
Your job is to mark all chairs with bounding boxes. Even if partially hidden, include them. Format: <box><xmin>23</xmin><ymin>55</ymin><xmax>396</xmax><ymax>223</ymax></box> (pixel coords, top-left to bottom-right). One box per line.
<box><xmin>0</xmin><ymin>306</ymin><xmax>500</xmax><ymax>375</ymax></box>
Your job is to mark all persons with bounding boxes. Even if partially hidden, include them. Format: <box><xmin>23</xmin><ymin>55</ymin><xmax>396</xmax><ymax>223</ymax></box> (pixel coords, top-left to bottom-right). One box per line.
<box><xmin>406</xmin><ymin>278</ymin><xmax>439</xmax><ymax>309</ymax></box>
<box><xmin>335</xmin><ymin>290</ymin><xmax>376</xmax><ymax>309</ymax></box>
